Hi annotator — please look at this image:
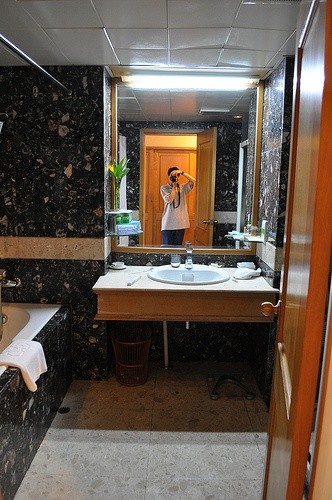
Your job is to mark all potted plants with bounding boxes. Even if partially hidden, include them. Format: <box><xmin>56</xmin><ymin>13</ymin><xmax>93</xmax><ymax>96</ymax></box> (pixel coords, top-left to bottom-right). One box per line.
<box><xmin>106</xmin><ymin>157</ymin><xmax>130</xmax><ymax>212</ymax></box>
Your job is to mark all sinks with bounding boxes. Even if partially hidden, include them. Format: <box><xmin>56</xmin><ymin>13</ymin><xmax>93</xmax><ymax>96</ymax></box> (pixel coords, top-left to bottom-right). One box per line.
<box><xmin>147</xmin><ymin>263</ymin><xmax>230</xmax><ymax>285</ymax></box>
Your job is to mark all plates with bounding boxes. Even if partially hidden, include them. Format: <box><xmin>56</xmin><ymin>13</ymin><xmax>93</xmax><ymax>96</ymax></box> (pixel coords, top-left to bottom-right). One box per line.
<box><xmin>109</xmin><ymin>265</ymin><xmax>126</xmax><ymax>269</ymax></box>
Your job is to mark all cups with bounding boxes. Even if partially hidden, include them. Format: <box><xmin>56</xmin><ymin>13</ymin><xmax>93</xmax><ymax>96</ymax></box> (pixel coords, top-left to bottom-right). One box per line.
<box><xmin>112</xmin><ymin>262</ymin><xmax>124</xmax><ymax>267</ymax></box>
<box><xmin>171</xmin><ymin>255</ymin><xmax>181</xmax><ymax>268</ymax></box>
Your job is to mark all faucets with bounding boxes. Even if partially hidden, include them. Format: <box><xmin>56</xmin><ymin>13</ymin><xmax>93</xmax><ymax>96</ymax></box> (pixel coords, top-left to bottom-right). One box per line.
<box><xmin>185</xmin><ymin>247</ymin><xmax>193</xmax><ymax>269</ymax></box>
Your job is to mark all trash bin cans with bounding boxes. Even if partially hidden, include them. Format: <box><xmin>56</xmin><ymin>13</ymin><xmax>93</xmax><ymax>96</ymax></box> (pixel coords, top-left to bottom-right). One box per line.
<box><xmin>112</xmin><ymin>323</ymin><xmax>153</xmax><ymax>386</ymax></box>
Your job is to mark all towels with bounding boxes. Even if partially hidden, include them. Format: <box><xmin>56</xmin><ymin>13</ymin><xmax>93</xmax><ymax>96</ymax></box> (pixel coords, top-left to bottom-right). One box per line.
<box><xmin>233</xmin><ymin>261</ymin><xmax>262</xmax><ymax>280</ymax></box>
<box><xmin>0</xmin><ymin>339</ymin><xmax>48</xmax><ymax>392</ymax></box>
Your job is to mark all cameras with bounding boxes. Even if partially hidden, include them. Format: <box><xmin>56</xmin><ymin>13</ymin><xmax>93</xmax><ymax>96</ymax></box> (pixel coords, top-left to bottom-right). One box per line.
<box><xmin>170</xmin><ymin>175</ymin><xmax>177</xmax><ymax>183</ymax></box>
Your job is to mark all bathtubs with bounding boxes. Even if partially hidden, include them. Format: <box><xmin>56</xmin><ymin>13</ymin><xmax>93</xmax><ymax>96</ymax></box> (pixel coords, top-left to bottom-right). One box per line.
<box><xmin>0</xmin><ymin>303</ymin><xmax>74</xmax><ymax>500</ymax></box>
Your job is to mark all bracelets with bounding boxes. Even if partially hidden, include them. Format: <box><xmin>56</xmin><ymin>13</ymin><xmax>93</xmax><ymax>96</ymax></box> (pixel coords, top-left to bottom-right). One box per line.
<box><xmin>181</xmin><ymin>171</ymin><xmax>184</xmax><ymax>175</ymax></box>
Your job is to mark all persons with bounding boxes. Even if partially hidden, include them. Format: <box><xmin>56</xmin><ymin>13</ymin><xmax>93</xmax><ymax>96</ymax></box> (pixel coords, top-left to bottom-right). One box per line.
<box><xmin>160</xmin><ymin>166</ymin><xmax>196</xmax><ymax>245</ymax></box>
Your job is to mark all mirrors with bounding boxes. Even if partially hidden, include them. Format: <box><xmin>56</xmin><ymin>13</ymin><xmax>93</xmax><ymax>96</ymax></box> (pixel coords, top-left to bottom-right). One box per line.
<box><xmin>111</xmin><ymin>77</ymin><xmax>264</xmax><ymax>255</ymax></box>
